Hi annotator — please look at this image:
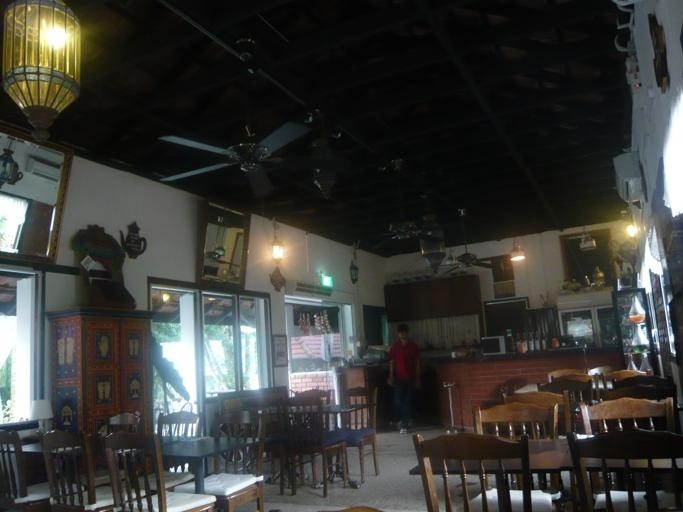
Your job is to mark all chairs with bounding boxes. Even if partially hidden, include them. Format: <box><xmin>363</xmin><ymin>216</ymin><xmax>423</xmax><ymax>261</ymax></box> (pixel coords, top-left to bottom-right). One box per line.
<box><xmin>412</xmin><ymin>364</ymin><xmax>683</xmax><ymax>511</ymax></box>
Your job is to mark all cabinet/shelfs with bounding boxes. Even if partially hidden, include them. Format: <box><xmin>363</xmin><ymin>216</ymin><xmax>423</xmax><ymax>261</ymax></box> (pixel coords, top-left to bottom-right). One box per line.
<box><xmin>339</xmin><ymin>365</ymin><xmax>388</xmax><ymax>429</ymax></box>
<box><xmin>45</xmin><ymin>308</ymin><xmax>156</xmax><ymax>473</ymax></box>
<box><xmin>383</xmin><ymin>274</ymin><xmax>480</xmax><ymax>320</ymax></box>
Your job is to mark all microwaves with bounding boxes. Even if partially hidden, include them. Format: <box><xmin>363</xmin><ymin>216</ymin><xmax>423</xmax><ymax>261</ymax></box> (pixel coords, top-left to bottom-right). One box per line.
<box><xmin>480</xmin><ymin>335</ymin><xmax>506</xmax><ymax>356</ymax></box>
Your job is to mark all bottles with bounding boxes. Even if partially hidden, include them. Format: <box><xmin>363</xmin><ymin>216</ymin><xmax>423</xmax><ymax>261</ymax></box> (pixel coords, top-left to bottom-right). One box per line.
<box><xmin>627</xmin><ymin>355</ymin><xmax>638</xmax><ymax>371</ymax></box>
<box><xmin>632</xmin><ymin>324</ymin><xmax>650</xmax><ymax>354</ymax></box>
<box><xmin>534</xmin><ymin>331</ymin><xmax>541</xmax><ymax>351</ymax></box>
<box><xmin>515</xmin><ymin>333</ymin><xmax>520</xmax><ymax>352</ymax></box>
<box><xmin>628</xmin><ymin>296</ymin><xmax>646</xmax><ymax>323</ymax></box>
<box><xmin>521</xmin><ymin>333</ymin><xmax>528</xmax><ymax>353</ymax></box>
<box><xmin>528</xmin><ymin>331</ymin><xmax>535</xmax><ymax>352</ymax></box>
<box><xmin>638</xmin><ymin>353</ymin><xmax>653</xmax><ymax>373</ymax></box>
<box><xmin>541</xmin><ymin>331</ymin><xmax>549</xmax><ymax>351</ymax></box>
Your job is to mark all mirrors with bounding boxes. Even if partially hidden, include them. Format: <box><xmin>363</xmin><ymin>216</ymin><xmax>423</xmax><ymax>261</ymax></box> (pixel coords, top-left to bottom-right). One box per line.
<box><xmin>196</xmin><ymin>204</ymin><xmax>252</xmax><ymax>289</ymax></box>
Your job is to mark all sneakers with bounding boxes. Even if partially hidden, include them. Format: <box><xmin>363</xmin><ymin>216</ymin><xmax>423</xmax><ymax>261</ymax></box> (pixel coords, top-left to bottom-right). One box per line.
<box><xmin>399</xmin><ymin>427</ymin><xmax>408</xmax><ymax>434</ymax></box>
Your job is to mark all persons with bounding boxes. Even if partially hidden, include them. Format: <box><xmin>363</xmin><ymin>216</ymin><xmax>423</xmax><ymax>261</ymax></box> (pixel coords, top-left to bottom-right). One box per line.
<box><xmin>388</xmin><ymin>324</ymin><xmax>421</xmax><ymax>434</ymax></box>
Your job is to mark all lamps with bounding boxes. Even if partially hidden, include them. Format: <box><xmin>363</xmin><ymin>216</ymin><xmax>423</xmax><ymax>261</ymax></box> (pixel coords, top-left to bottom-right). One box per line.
<box><xmin>271</xmin><ymin>219</ymin><xmax>285</xmax><ymax>266</ymax></box>
<box><xmin>0</xmin><ymin>0</ymin><xmax>83</xmax><ymax>147</ymax></box>
<box><xmin>351</xmin><ymin>243</ymin><xmax>360</xmax><ymax>284</ymax></box>
<box><xmin>509</xmin><ymin>240</ymin><xmax>526</xmax><ymax>262</ymax></box>
<box><xmin>0</xmin><ymin>148</ymin><xmax>23</xmax><ymax>190</ymax></box>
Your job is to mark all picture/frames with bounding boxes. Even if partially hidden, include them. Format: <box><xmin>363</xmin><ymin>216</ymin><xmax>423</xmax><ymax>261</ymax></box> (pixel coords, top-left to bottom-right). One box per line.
<box><xmin>272</xmin><ymin>334</ymin><xmax>287</xmax><ymax>367</ymax></box>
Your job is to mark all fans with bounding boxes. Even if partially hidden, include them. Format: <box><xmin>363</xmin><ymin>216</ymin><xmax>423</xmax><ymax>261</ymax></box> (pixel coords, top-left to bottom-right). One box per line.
<box><xmin>566</xmin><ymin>232</ymin><xmax>599</xmax><ymax>252</ymax></box>
<box><xmin>155</xmin><ymin>114</ymin><xmax>313</xmax><ymax>184</ymax></box>
<box><xmin>440</xmin><ymin>252</ymin><xmax>508</xmax><ymax>274</ymax></box>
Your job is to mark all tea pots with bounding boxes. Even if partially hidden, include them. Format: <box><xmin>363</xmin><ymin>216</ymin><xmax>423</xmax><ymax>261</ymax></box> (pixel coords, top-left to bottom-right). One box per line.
<box><xmin>118</xmin><ymin>222</ymin><xmax>147</xmax><ymax>260</ymax></box>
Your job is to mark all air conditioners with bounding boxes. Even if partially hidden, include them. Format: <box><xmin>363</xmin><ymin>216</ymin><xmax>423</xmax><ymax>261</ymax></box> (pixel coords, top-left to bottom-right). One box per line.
<box><xmin>286</xmin><ymin>276</ymin><xmax>334</xmax><ymax>300</ymax></box>
